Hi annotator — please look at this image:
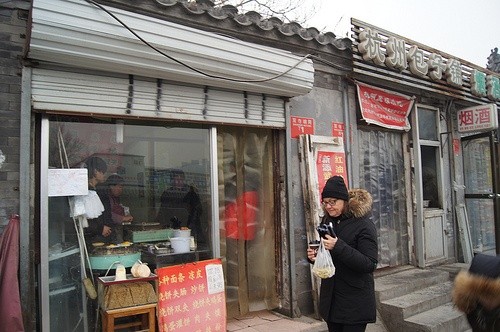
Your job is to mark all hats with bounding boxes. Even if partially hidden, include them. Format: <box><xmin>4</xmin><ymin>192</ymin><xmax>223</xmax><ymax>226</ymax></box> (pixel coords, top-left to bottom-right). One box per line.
<box><xmin>321</xmin><ymin>176</ymin><xmax>350</xmax><ymax>201</ymax></box>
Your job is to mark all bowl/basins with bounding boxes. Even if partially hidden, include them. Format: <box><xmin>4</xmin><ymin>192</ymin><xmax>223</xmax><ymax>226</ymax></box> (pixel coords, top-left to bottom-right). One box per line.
<box><xmin>173</xmin><ymin>229</ymin><xmax>192</xmax><ymax>237</ymax></box>
<box><xmin>170</xmin><ymin>237</ymin><xmax>191</xmax><ymax>254</ymax></box>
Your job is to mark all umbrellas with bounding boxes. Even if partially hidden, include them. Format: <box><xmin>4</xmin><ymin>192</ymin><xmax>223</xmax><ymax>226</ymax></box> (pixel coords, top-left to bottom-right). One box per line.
<box><xmin>0</xmin><ymin>214</ymin><xmax>26</xmax><ymax>332</ymax></box>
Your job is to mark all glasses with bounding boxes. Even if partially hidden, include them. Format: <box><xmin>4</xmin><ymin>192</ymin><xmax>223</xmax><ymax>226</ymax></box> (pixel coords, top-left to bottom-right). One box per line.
<box><xmin>321</xmin><ymin>199</ymin><xmax>339</xmax><ymax>205</ymax></box>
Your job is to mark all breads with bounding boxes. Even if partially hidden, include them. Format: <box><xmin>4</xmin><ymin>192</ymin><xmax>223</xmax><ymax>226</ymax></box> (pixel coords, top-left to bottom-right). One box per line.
<box><xmin>130</xmin><ymin>264</ymin><xmax>151</xmax><ymax>278</ymax></box>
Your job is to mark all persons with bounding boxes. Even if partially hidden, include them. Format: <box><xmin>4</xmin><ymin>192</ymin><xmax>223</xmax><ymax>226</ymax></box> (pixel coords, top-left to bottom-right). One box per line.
<box><xmin>84</xmin><ymin>157</ymin><xmax>116</xmax><ymax>246</ymax></box>
<box><xmin>104</xmin><ymin>175</ymin><xmax>133</xmax><ymax>242</ymax></box>
<box><xmin>156</xmin><ymin>169</ymin><xmax>201</xmax><ymax>264</ymax></box>
<box><xmin>220</xmin><ymin>162</ymin><xmax>258</xmax><ymax>298</ymax></box>
<box><xmin>453</xmin><ymin>255</ymin><xmax>500</xmax><ymax>332</ymax></box>
<box><xmin>307</xmin><ymin>176</ymin><xmax>378</xmax><ymax>332</ymax></box>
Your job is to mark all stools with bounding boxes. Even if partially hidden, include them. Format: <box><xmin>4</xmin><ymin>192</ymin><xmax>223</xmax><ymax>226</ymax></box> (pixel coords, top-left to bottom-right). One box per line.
<box><xmin>101</xmin><ymin>303</ymin><xmax>157</xmax><ymax>332</ymax></box>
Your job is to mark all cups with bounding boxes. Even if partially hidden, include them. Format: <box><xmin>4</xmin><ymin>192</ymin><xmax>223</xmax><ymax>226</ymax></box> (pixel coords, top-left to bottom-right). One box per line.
<box><xmin>309</xmin><ymin>240</ymin><xmax>320</xmax><ymax>260</ymax></box>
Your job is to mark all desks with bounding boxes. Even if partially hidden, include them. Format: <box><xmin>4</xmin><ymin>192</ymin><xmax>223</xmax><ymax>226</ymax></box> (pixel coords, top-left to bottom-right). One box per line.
<box><xmin>142</xmin><ymin>247</ymin><xmax>209</xmax><ymax>268</ymax></box>
